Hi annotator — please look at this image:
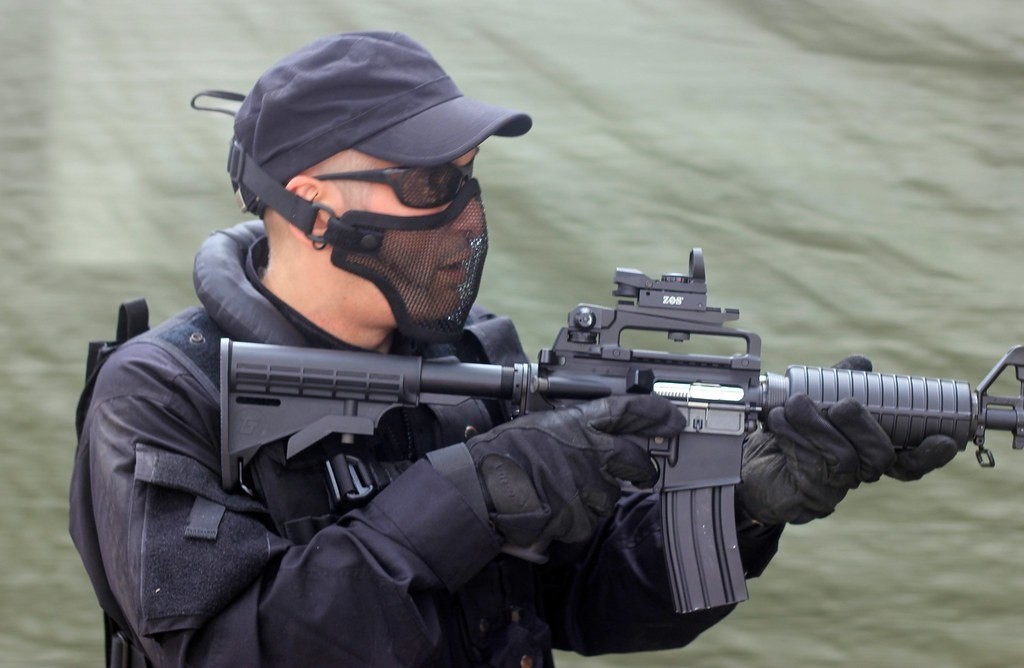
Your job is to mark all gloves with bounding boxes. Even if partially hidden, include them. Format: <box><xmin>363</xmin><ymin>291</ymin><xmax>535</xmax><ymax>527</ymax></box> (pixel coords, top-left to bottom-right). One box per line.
<box><xmin>730</xmin><ymin>355</ymin><xmax>960</xmax><ymax>528</ymax></box>
<box><xmin>470</xmin><ymin>386</ymin><xmax>685</xmax><ymax>545</ymax></box>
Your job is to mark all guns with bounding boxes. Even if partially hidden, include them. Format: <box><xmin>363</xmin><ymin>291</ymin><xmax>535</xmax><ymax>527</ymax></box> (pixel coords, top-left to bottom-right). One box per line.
<box><xmin>218</xmin><ymin>245</ymin><xmax>1020</xmax><ymax>614</ymax></box>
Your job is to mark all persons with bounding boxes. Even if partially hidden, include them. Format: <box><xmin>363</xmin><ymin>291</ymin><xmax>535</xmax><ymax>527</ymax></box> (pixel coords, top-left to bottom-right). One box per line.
<box><xmin>67</xmin><ymin>33</ymin><xmax>956</xmax><ymax>668</ymax></box>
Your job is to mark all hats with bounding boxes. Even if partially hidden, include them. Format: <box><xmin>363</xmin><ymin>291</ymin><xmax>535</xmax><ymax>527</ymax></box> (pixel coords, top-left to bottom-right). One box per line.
<box><xmin>226</xmin><ymin>30</ymin><xmax>533</xmax><ymax>214</ymax></box>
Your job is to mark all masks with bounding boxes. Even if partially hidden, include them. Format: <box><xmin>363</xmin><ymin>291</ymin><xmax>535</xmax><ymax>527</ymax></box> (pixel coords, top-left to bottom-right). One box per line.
<box><xmin>229</xmin><ymin>140</ymin><xmax>491</xmax><ymax>343</ymax></box>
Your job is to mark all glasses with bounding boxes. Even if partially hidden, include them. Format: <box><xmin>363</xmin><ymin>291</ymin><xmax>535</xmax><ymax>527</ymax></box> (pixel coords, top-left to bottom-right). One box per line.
<box><xmin>312</xmin><ymin>147</ymin><xmax>483</xmax><ymax>208</ymax></box>
